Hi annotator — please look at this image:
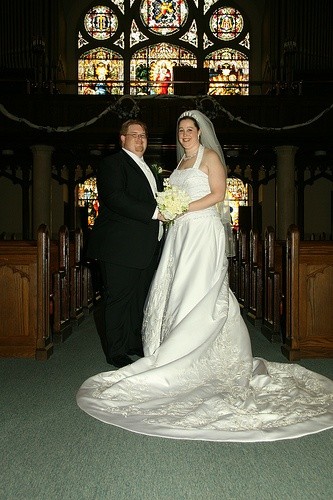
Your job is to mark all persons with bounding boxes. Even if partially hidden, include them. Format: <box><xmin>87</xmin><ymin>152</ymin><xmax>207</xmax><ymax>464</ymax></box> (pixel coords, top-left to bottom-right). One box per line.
<box><xmin>76</xmin><ymin>110</ymin><xmax>333</xmax><ymax>443</ymax></box>
<box><xmin>80</xmin><ymin>119</ymin><xmax>169</xmax><ymax>368</ymax></box>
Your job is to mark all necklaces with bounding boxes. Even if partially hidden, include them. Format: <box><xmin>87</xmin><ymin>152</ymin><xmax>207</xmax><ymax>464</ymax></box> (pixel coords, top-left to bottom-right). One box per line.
<box><xmin>183</xmin><ymin>153</ymin><xmax>197</xmax><ymax>161</ymax></box>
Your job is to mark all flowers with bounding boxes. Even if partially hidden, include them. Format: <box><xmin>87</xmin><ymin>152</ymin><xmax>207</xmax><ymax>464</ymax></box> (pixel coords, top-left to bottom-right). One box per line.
<box><xmin>152</xmin><ymin>164</ymin><xmax>163</xmax><ymax>178</ymax></box>
<box><xmin>156</xmin><ymin>177</ymin><xmax>190</xmax><ymax>233</ymax></box>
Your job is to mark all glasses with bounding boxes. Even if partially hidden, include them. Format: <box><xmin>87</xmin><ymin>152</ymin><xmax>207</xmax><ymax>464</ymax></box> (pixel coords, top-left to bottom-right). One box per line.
<box><xmin>125</xmin><ymin>133</ymin><xmax>147</xmax><ymax>138</ymax></box>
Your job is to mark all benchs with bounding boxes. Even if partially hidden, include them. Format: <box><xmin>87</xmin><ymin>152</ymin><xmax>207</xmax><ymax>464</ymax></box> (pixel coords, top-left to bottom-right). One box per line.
<box><xmin>0</xmin><ymin>225</ymin><xmax>101</xmax><ymax>362</ymax></box>
<box><xmin>228</xmin><ymin>225</ymin><xmax>333</xmax><ymax>360</ymax></box>
